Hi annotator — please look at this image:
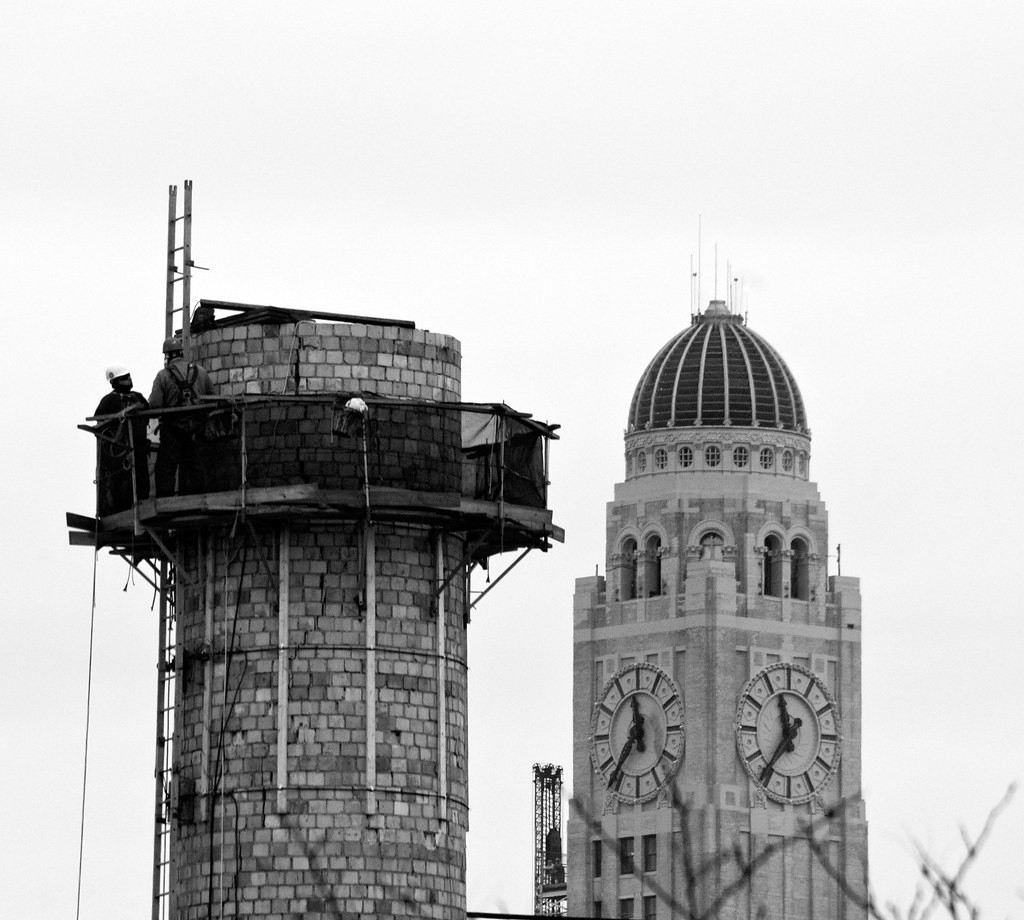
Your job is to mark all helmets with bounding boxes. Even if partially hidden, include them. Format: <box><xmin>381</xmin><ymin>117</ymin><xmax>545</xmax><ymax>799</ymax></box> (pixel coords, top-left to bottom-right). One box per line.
<box><xmin>163</xmin><ymin>337</ymin><xmax>183</xmax><ymax>352</ymax></box>
<box><xmin>106</xmin><ymin>365</ymin><xmax>130</xmax><ymax>381</ymax></box>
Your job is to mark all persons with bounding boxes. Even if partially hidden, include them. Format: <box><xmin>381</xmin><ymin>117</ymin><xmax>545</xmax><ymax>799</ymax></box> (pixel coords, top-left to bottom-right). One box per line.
<box><xmin>148</xmin><ymin>336</ymin><xmax>216</xmax><ymax>499</ymax></box>
<box><xmin>542</xmin><ymin>858</ymin><xmax>565</xmax><ymax>884</ymax></box>
<box><xmin>94</xmin><ymin>365</ymin><xmax>149</xmax><ymax>519</ymax></box>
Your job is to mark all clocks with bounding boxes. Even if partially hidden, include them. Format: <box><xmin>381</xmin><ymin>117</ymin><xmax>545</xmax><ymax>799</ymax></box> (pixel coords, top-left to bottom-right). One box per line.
<box><xmin>735</xmin><ymin>661</ymin><xmax>844</xmax><ymax>805</ymax></box>
<box><xmin>589</xmin><ymin>662</ymin><xmax>687</xmax><ymax>806</ymax></box>
